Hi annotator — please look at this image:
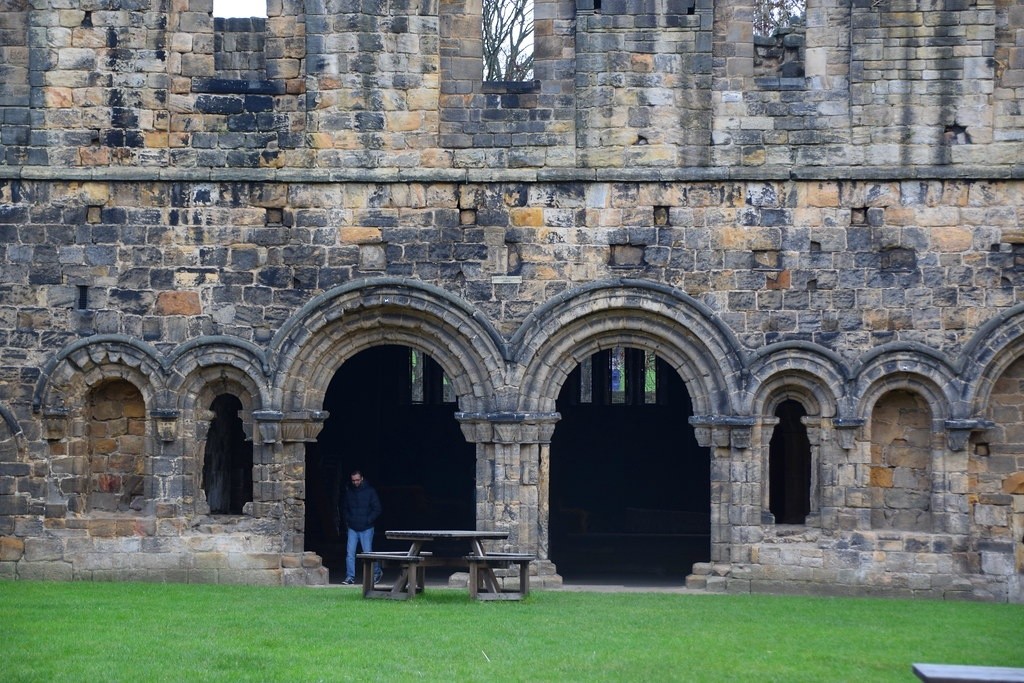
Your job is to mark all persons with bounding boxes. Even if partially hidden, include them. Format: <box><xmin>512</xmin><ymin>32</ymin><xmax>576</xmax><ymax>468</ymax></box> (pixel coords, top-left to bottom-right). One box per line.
<box><xmin>339</xmin><ymin>468</ymin><xmax>383</xmax><ymax>586</ymax></box>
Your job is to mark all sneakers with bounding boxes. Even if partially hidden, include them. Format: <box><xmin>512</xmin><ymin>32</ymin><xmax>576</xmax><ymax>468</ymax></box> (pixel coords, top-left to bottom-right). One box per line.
<box><xmin>373</xmin><ymin>571</ymin><xmax>383</xmax><ymax>584</ymax></box>
<box><xmin>342</xmin><ymin>577</ymin><xmax>355</xmax><ymax>584</ymax></box>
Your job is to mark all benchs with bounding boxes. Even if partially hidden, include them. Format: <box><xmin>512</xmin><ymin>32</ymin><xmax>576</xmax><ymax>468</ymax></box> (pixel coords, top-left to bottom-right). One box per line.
<box><xmin>466</xmin><ymin>550</ymin><xmax>536</xmax><ymax>602</ymax></box>
<box><xmin>357</xmin><ymin>550</ymin><xmax>434</xmax><ymax>602</ymax></box>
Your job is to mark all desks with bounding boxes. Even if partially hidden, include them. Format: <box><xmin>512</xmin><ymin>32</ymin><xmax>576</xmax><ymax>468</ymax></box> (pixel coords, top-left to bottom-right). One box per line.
<box><xmin>383</xmin><ymin>529</ymin><xmax>509</xmax><ymax>594</ymax></box>
